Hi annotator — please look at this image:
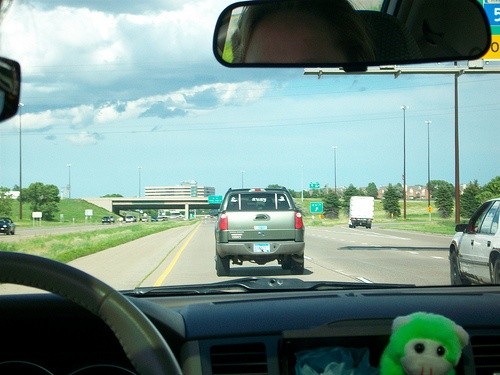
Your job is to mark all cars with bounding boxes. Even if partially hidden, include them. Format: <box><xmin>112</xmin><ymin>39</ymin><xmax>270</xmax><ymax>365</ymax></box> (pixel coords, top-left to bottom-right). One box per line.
<box><xmin>101</xmin><ymin>217</ymin><xmax>111</xmax><ymax>223</ymax></box>
<box><xmin>0</xmin><ymin>217</ymin><xmax>16</xmax><ymax>235</ymax></box>
<box><xmin>141</xmin><ymin>217</ymin><xmax>169</xmax><ymax>222</ymax></box>
<box><xmin>125</xmin><ymin>215</ymin><xmax>137</xmax><ymax>223</ymax></box>
<box><xmin>110</xmin><ymin>216</ymin><xmax>115</xmax><ymax>223</ymax></box>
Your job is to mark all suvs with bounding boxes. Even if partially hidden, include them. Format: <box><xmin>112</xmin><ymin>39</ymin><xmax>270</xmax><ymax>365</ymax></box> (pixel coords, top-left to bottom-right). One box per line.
<box><xmin>448</xmin><ymin>198</ymin><xmax>500</xmax><ymax>286</ymax></box>
<box><xmin>215</xmin><ymin>186</ymin><xmax>305</xmax><ymax>275</ymax></box>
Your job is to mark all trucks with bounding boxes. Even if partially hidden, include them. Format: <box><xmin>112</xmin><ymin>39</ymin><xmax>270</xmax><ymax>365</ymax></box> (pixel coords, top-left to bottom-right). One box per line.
<box><xmin>348</xmin><ymin>195</ymin><xmax>374</xmax><ymax>230</ymax></box>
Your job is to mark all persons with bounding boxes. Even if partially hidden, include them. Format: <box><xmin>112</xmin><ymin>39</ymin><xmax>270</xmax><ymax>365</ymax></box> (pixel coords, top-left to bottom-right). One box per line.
<box><xmin>232</xmin><ymin>0</ymin><xmax>376</xmax><ymax>64</ymax></box>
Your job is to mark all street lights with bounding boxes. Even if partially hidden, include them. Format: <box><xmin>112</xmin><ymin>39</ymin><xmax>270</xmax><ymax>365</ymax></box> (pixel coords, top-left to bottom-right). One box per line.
<box><xmin>332</xmin><ymin>145</ymin><xmax>339</xmax><ymax>192</ymax></box>
<box><xmin>67</xmin><ymin>164</ymin><xmax>72</xmax><ymax>198</ymax></box>
<box><xmin>138</xmin><ymin>166</ymin><xmax>142</xmax><ymax>198</ymax></box>
<box><xmin>424</xmin><ymin>120</ymin><xmax>433</xmax><ymax>222</ymax></box>
<box><xmin>400</xmin><ymin>104</ymin><xmax>409</xmax><ymax>219</ymax></box>
<box><xmin>17</xmin><ymin>102</ymin><xmax>24</xmax><ymax>219</ymax></box>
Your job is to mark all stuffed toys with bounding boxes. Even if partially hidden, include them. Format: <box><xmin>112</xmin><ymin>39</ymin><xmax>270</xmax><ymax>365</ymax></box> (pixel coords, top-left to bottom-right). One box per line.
<box><xmin>380</xmin><ymin>312</ymin><xmax>470</xmax><ymax>375</ymax></box>
